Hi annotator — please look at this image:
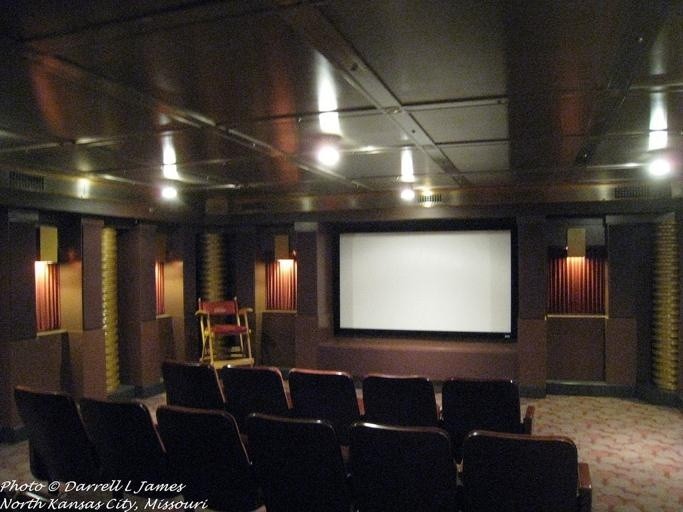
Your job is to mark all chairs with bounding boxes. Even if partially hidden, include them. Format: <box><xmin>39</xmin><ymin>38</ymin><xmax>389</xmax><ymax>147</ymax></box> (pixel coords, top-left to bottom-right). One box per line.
<box><xmin>0</xmin><ymin>296</ymin><xmax>592</xmax><ymax>512</ymax></box>
